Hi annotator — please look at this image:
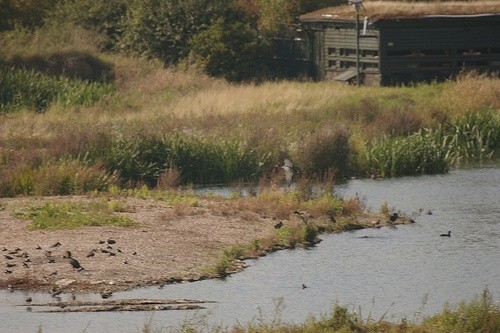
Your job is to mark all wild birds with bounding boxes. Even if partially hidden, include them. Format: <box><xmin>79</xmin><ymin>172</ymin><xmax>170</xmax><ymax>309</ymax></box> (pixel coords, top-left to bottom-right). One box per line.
<box><xmin>2</xmin><ymin>237</ymin><xmax>137</xmax><ymax>304</ymax></box>
<box><xmin>272</xmin><ymin>203</ymin><xmax>452</xmax><ymax>239</ymax></box>
<box><xmin>301</xmin><ymin>283</ymin><xmax>307</xmax><ymax>289</ymax></box>
